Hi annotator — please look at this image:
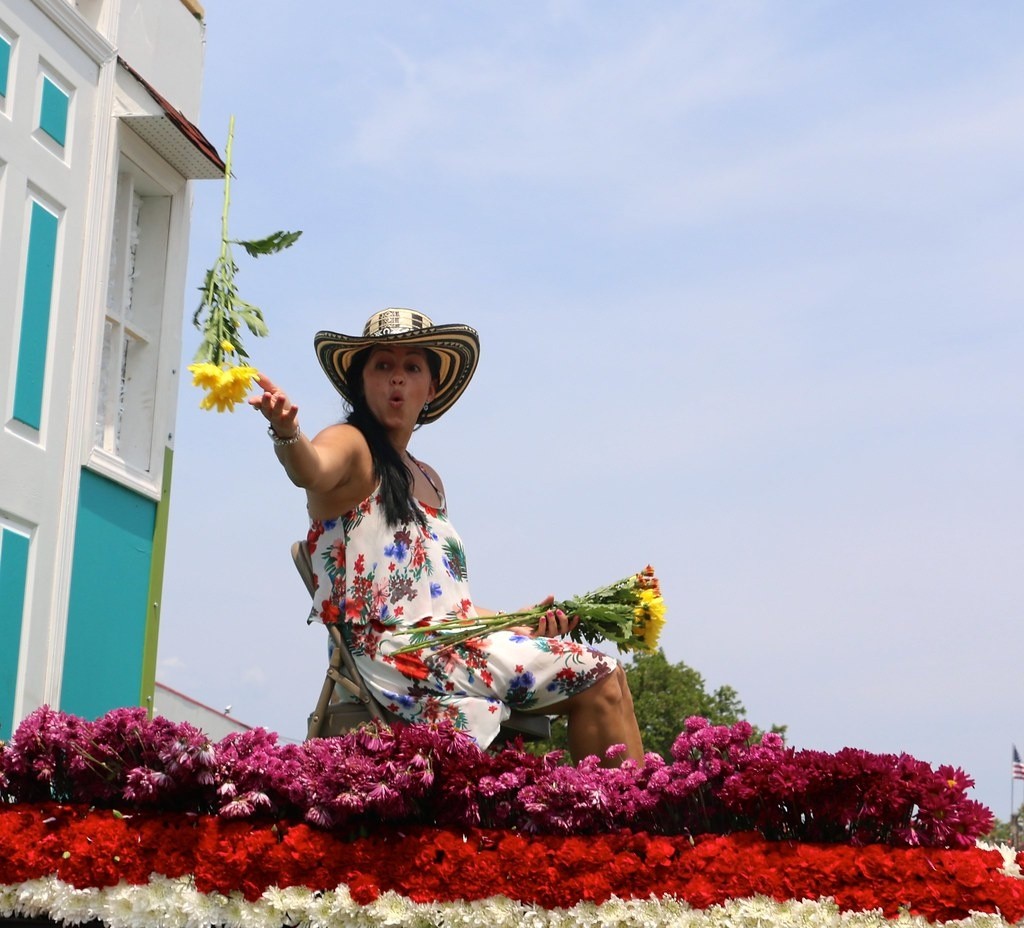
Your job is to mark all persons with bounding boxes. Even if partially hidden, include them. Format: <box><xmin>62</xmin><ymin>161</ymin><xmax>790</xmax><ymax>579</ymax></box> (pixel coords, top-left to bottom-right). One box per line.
<box><xmin>241</xmin><ymin>308</ymin><xmax>649</xmax><ymax>779</ymax></box>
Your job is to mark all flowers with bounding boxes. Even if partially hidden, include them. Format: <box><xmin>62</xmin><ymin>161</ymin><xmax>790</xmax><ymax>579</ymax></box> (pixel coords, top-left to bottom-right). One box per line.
<box><xmin>391</xmin><ymin>565</ymin><xmax>666</xmax><ymax>667</ymax></box>
<box><xmin>184</xmin><ymin>116</ymin><xmax>305</xmax><ymax>414</ymax></box>
<box><xmin>2</xmin><ymin>705</ymin><xmax>1024</xmax><ymax>926</ymax></box>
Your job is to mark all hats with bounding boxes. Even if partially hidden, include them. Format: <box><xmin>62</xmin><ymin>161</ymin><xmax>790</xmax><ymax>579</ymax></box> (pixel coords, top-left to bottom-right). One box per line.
<box><xmin>313</xmin><ymin>308</ymin><xmax>480</xmax><ymax>426</ymax></box>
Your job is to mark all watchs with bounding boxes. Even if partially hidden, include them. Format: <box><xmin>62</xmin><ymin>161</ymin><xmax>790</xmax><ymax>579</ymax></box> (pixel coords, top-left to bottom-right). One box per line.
<box><xmin>268</xmin><ymin>423</ymin><xmax>302</xmax><ymax>447</ymax></box>
